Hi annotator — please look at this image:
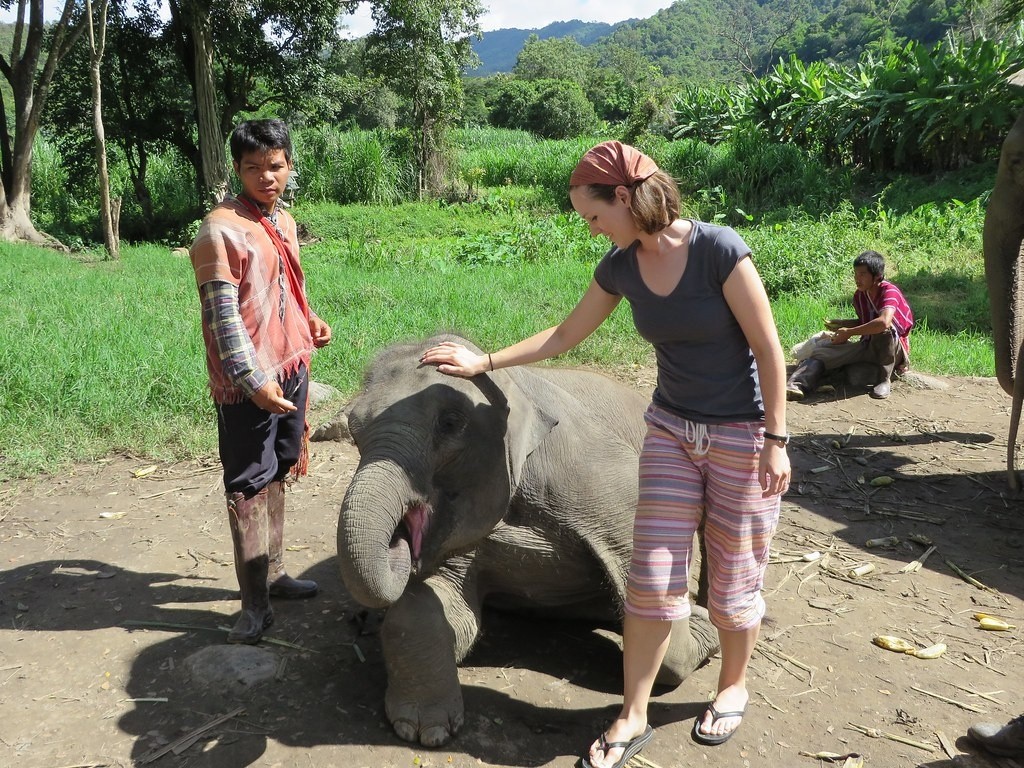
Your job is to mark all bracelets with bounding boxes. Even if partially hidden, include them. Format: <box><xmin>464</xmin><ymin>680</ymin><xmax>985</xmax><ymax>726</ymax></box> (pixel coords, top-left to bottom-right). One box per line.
<box><xmin>488</xmin><ymin>351</ymin><xmax>495</xmax><ymax>373</ymax></box>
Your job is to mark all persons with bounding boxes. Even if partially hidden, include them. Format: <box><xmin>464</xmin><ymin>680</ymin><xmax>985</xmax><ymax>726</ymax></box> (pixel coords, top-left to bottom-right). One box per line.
<box><xmin>785</xmin><ymin>250</ymin><xmax>914</xmax><ymax>399</ymax></box>
<box><xmin>419</xmin><ymin>141</ymin><xmax>791</xmax><ymax>768</ymax></box>
<box><xmin>191</xmin><ymin>120</ymin><xmax>333</xmax><ymax>647</ymax></box>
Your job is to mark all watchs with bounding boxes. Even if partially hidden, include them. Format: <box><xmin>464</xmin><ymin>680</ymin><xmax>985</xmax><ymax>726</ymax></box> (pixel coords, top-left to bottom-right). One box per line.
<box><xmin>763</xmin><ymin>431</ymin><xmax>793</xmax><ymax>446</ymax></box>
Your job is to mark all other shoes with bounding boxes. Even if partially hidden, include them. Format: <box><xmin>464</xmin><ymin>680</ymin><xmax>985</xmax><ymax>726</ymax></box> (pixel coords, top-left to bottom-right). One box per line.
<box><xmin>965</xmin><ymin>714</ymin><xmax>1024</xmax><ymax>759</ymax></box>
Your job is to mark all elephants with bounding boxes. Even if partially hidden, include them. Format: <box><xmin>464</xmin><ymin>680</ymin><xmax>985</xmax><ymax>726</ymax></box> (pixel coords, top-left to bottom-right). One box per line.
<box><xmin>982</xmin><ymin>111</ymin><xmax>1024</xmax><ymax>502</ymax></box>
<box><xmin>307</xmin><ymin>332</ymin><xmax>723</xmax><ymax>748</ymax></box>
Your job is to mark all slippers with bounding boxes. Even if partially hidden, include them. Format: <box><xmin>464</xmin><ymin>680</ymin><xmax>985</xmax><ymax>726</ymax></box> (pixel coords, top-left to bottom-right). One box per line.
<box><xmin>693</xmin><ymin>700</ymin><xmax>749</xmax><ymax>744</ymax></box>
<box><xmin>581</xmin><ymin>723</ymin><xmax>654</xmax><ymax>768</ymax></box>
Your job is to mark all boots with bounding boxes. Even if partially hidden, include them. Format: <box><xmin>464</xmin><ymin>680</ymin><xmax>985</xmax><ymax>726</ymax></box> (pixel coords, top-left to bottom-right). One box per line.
<box><xmin>869</xmin><ymin>331</ymin><xmax>896</xmax><ymax>400</ymax></box>
<box><xmin>786</xmin><ymin>357</ymin><xmax>825</xmax><ymax>401</ymax></box>
<box><xmin>266</xmin><ymin>480</ymin><xmax>319</xmax><ymax>600</ymax></box>
<box><xmin>223</xmin><ymin>487</ymin><xmax>274</xmax><ymax>645</ymax></box>
